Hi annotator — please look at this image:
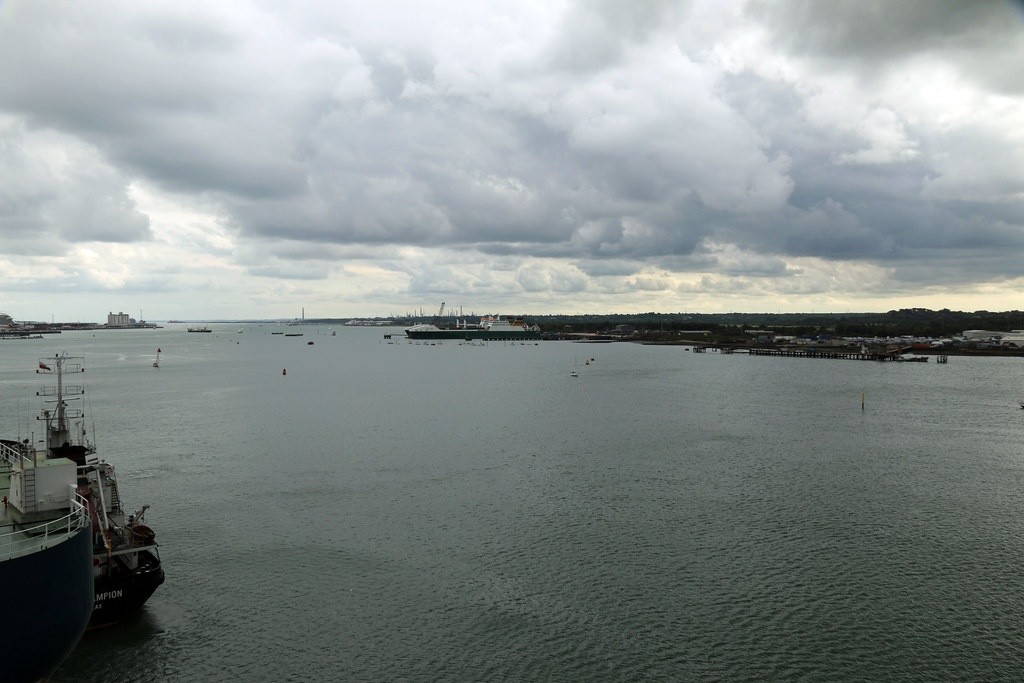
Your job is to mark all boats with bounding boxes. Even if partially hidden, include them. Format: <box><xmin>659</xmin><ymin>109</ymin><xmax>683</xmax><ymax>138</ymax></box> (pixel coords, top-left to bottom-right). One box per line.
<box><xmin>188</xmin><ymin>328</ymin><xmax>212</xmax><ymax>332</ymax></box>
<box><xmin>571</xmin><ymin>371</ymin><xmax>578</xmax><ymax>377</ymax></box>
<box><xmin>286</xmin><ymin>333</ymin><xmax>303</xmax><ymax>336</ymax></box>
<box><xmin>405</xmin><ymin>317</ymin><xmax>542</xmax><ymax>339</ymax></box>
<box><xmin>271</xmin><ymin>332</ymin><xmax>283</xmax><ymax>334</ymax></box>
<box><xmin>895</xmin><ymin>357</ymin><xmax>929</xmax><ymax>361</ymax></box>
<box><xmin>0</xmin><ymin>351</ymin><xmax>164</xmax><ymax>683</ymax></box>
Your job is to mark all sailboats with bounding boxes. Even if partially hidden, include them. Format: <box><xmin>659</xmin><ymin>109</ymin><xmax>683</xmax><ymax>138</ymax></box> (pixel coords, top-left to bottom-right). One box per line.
<box><xmin>153</xmin><ymin>349</ymin><xmax>162</xmax><ymax>367</ymax></box>
